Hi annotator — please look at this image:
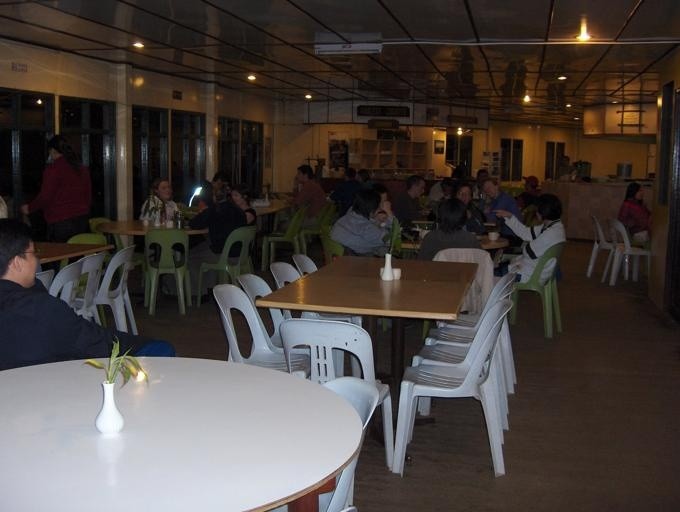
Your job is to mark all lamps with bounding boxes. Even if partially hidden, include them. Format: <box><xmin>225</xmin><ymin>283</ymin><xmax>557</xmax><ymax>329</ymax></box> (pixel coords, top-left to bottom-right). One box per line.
<box><xmin>314</xmin><ymin>43</ymin><xmax>383</xmax><ymax>55</ymax></box>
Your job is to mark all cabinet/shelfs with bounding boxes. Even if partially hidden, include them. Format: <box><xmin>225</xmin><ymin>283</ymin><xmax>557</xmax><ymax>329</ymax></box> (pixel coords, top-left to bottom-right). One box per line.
<box><xmin>350</xmin><ymin>137</ymin><xmax>428</xmax><ymax>172</ymax></box>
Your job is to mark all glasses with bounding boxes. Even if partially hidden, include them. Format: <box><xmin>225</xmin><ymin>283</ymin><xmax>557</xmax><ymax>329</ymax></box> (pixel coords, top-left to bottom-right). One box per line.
<box><xmin>23</xmin><ymin>247</ymin><xmax>41</xmax><ymax>257</ymax></box>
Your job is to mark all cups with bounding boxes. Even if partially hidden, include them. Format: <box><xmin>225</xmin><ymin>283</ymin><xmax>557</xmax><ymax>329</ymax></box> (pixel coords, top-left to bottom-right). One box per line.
<box><xmin>540</xmin><ymin>173</ymin><xmax>577</xmax><ymax>183</ymax></box>
<box><xmin>425</xmin><ymin>168</ymin><xmax>435</xmax><ymax>181</ymax></box>
<box><xmin>379</xmin><ymin>267</ymin><xmax>402</xmax><ymax>280</ymax></box>
<box><xmin>488</xmin><ymin>232</ymin><xmax>500</xmax><ymax>241</ymax></box>
<box><xmin>617</xmin><ymin>163</ymin><xmax>632</xmax><ymax>177</ymax></box>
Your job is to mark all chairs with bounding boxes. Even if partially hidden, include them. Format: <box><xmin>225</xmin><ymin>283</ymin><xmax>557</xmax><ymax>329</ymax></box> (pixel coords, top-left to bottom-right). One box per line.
<box><xmin>586</xmin><ymin>216</ymin><xmax>629</xmax><ymax>281</ymax></box>
<box><xmin>599</xmin><ymin>218</ymin><xmax>648</xmax><ymax>286</ymax></box>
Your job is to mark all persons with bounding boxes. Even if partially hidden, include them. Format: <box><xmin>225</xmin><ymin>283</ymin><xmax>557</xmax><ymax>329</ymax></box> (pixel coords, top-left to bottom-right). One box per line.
<box><xmin>616</xmin><ymin>184</ymin><xmax>652</xmax><ymax>242</ymax></box>
<box><xmin>22</xmin><ymin>134</ymin><xmax>92</xmax><ymax>279</ymax></box>
<box><xmin>0</xmin><ymin>218</ymin><xmax>176</xmax><ymax>371</ymax></box>
<box><xmin>286</xmin><ymin>163</ymin><xmax>370</xmax><ymax>228</ymax></box>
<box><xmin>329</xmin><ymin>161</ymin><xmax>566</xmax><ymax>328</ymax></box>
<box><xmin>138</xmin><ymin>171</ymin><xmax>257</xmax><ymax>297</ymax></box>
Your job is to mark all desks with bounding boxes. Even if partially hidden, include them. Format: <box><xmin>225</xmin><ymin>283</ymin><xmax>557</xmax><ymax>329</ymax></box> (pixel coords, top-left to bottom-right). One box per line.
<box><xmin>545</xmin><ymin>180</ymin><xmax>653</xmax><ymax>242</ymax></box>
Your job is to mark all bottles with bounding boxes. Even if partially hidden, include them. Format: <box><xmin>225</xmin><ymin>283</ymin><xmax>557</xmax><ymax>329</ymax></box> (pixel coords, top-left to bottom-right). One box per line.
<box><xmin>249</xmin><ymin>191</ymin><xmax>270</xmax><ymax>209</ymax></box>
<box><xmin>142</xmin><ymin>210</ymin><xmax>185</xmax><ymax>229</ymax></box>
<box><xmin>412</xmin><ymin>223</ymin><xmax>430</xmax><ymax>241</ymax></box>
